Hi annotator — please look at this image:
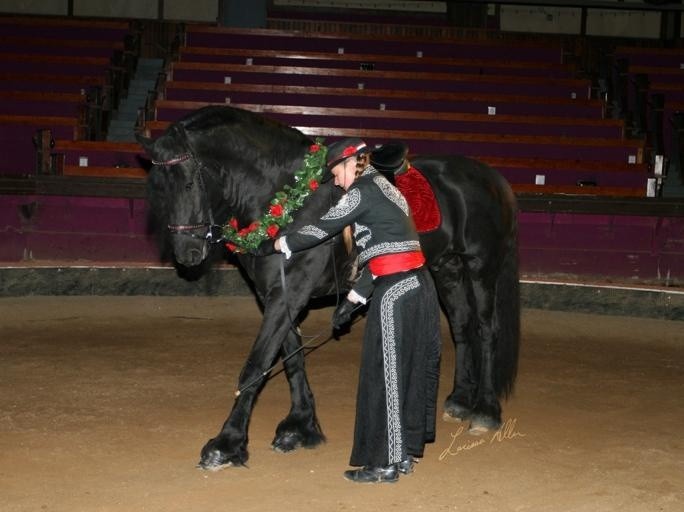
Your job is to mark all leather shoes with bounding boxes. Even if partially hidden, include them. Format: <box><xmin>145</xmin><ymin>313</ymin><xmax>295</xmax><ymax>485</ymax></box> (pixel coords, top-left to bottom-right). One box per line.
<box><xmin>344</xmin><ymin>456</ymin><xmax>416</xmax><ymax>484</ymax></box>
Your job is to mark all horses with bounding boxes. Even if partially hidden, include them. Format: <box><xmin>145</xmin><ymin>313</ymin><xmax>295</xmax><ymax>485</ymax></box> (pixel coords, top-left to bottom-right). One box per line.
<box><xmin>133</xmin><ymin>101</ymin><xmax>522</xmax><ymax>473</ymax></box>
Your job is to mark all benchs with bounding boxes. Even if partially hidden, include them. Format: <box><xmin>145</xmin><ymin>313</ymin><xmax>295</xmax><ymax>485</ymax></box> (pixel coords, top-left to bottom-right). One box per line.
<box><xmin>5</xmin><ymin>16</ymin><xmax>680</xmax><ymax>285</ymax></box>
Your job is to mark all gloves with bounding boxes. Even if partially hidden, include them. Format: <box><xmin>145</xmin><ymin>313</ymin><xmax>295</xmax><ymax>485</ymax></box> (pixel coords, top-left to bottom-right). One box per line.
<box><xmin>254</xmin><ymin>238</ymin><xmax>276</xmax><ymax>257</ymax></box>
<box><xmin>331</xmin><ymin>299</ymin><xmax>359</xmax><ymax>331</ymax></box>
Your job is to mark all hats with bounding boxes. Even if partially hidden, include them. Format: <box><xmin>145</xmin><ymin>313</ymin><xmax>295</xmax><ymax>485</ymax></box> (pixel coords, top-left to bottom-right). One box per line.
<box><xmin>318</xmin><ymin>136</ymin><xmax>369</xmax><ymax>184</ymax></box>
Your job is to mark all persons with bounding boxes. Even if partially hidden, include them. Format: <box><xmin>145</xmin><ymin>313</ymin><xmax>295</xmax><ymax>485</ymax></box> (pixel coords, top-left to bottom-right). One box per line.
<box><xmin>248</xmin><ymin>138</ymin><xmax>443</xmax><ymax>484</ymax></box>
